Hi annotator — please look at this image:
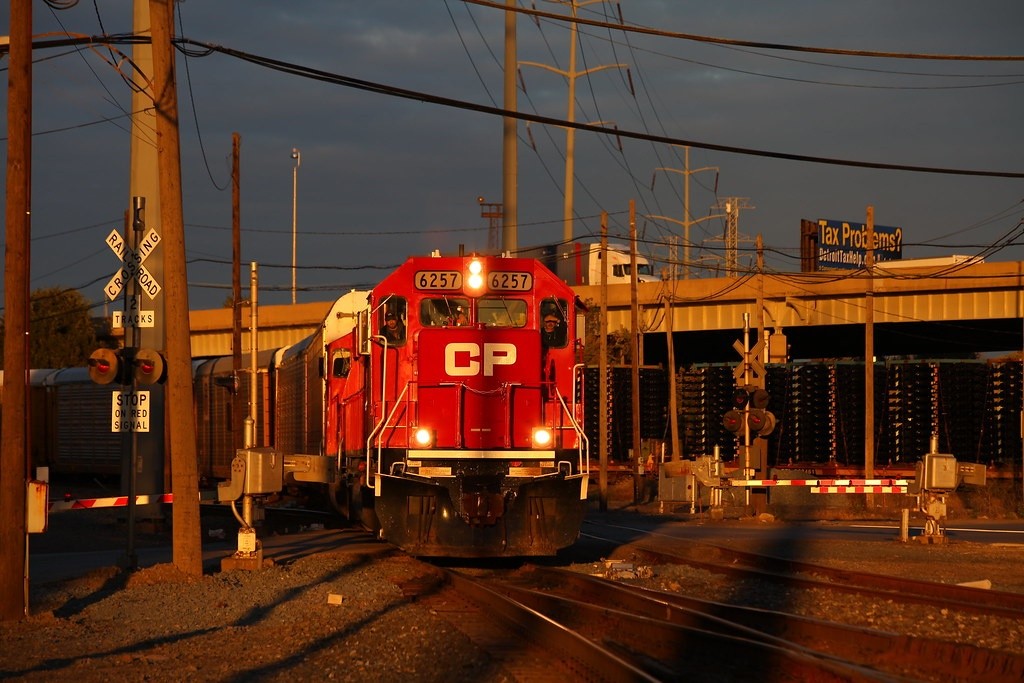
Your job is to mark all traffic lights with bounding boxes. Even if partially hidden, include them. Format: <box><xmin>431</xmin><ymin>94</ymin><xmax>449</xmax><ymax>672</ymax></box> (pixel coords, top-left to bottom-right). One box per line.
<box><xmin>722</xmin><ymin>411</ymin><xmax>742</xmax><ymax>432</ymax></box>
<box><xmin>86</xmin><ymin>348</ymin><xmax>119</xmax><ymax>387</ymax></box>
<box><xmin>748</xmin><ymin>411</ymin><xmax>766</xmax><ymax>431</ymax></box>
<box><xmin>132</xmin><ymin>349</ymin><xmax>164</xmax><ymax>384</ymax></box>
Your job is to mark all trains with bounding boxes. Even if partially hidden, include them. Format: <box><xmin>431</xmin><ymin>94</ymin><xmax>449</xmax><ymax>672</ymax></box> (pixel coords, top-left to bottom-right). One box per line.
<box><xmin>1</xmin><ymin>245</ymin><xmax>593</xmax><ymax>560</ymax></box>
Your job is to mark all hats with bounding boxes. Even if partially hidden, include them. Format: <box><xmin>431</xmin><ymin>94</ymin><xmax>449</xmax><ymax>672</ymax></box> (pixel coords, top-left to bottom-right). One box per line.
<box><xmin>545</xmin><ymin>315</ymin><xmax>557</xmax><ymax>321</ymax></box>
<box><xmin>385</xmin><ymin>311</ymin><xmax>396</xmax><ymax>318</ymax></box>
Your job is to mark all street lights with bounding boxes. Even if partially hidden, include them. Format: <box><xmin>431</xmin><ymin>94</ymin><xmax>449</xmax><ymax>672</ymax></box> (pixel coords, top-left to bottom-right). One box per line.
<box><xmin>289</xmin><ymin>146</ymin><xmax>301</xmax><ymax>306</ymax></box>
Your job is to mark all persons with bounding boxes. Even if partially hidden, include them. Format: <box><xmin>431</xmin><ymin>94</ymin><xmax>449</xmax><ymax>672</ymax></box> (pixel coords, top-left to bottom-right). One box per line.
<box><xmin>433</xmin><ymin>300</ymin><xmax>468</xmax><ymax>326</ymax></box>
<box><xmin>541</xmin><ymin>315</ymin><xmax>565</xmax><ymax>349</ymax></box>
<box><xmin>380</xmin><ymin>309</ymin><xmax>406</xmax><ymax>343</ymax></box>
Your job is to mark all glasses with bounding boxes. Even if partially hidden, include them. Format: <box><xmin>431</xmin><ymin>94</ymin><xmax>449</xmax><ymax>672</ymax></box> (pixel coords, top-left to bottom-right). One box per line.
<box><xmin>386</xmin><ymin>316</ymin><xmax>395</xmax><ymax>321</ymax></box>
